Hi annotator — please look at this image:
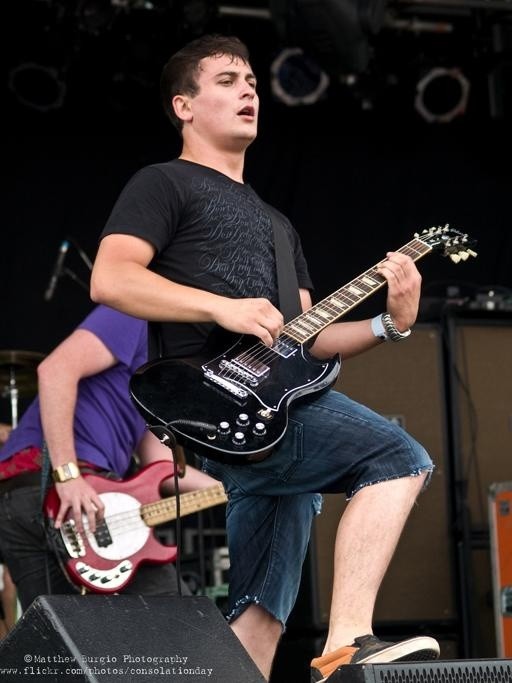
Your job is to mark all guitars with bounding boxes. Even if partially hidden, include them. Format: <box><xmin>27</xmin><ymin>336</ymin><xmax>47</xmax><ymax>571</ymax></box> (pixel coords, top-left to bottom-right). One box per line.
<box><xmin>44</xmin><ymin>461</ymin><xmax>229</xmax><ymax>593</ymax></box>
<box><xmin>129</xmin><ymin>223</ymin><xmax>478</xmax><ymax>465</ymax></box>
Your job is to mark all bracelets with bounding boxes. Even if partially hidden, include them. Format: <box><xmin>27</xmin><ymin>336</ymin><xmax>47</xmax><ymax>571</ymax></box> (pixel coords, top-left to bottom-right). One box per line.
<box><xmin>383</xmin><ymin>313</ymin><xmax>411</xmax><ymax>341</ymax></box>
<box><xmin>50</xmin><ymin>459</ymin><xmax>82</xmax><ymax>484</ymax></box>
<box><xmin>371</xmin><ymin>313</ymin><xmax>391</xmax><ymax>343</ymax></box>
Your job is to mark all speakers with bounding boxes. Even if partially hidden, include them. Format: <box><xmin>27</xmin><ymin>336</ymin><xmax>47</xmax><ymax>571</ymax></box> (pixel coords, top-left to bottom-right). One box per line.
<box><xmin>0</xmin><ymin>595</ymin><xmax>269</xmax><ymax>682</ymax></box>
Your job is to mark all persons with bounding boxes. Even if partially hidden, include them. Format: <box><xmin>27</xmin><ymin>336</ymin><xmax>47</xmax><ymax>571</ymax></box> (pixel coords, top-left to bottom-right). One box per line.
<box><xmin>86</xmin><ymin>28</ymin><xmax>445</xmax><ymax>681</ymax></box>
<box><xmin>1</xmin><ymin>304</ymin><xmax>223</xmax><ymax>610</ymax></box>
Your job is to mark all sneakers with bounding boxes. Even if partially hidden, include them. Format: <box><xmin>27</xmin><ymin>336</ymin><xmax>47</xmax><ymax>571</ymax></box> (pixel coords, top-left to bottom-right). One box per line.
<box><xmin>310</xmin><ymin>634</ymin><xmax>441</xmax><ymax>683</ymax></box>
